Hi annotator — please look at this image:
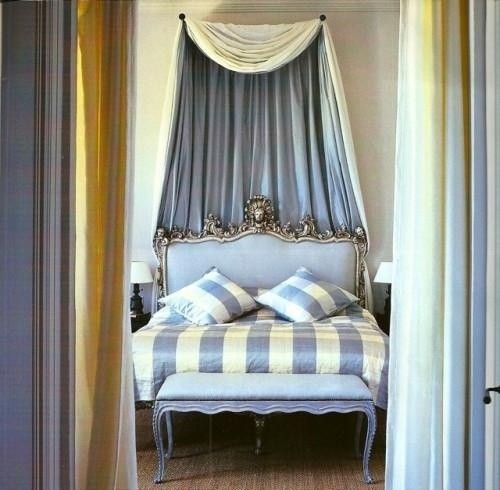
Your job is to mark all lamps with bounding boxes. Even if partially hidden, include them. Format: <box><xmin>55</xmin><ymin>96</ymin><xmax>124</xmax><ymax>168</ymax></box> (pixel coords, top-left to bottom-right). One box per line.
<box><xmin>129</xmin><ymin>261</ymin><xmax>153</xmax><ymax>315</ymax></box>
<box><xmin>373</xmin><ymin>261</ymin><xmax>394</xmax><ymax>314</ymax></box>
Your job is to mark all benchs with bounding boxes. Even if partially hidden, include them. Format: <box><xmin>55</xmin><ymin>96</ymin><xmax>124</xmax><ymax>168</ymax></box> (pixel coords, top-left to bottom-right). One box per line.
<box><xmin>154</xmin><ymin>372</ymin><xmax>378</xmax><ymax>483</ymax></box>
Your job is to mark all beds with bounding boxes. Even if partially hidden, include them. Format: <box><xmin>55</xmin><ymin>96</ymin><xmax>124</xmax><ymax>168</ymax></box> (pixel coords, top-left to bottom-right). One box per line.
<box><xmin>132</xmin><ymin>196</ymin><xmax>389</xmax><ymax>459</ymax></box>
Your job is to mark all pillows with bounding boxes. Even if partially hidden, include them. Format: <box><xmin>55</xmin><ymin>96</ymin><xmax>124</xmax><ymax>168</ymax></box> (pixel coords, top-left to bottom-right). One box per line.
<box><xmin>154</xmin><ymin>266</ymin><xmax>362</xmax><ymax>326</ymax></box>
